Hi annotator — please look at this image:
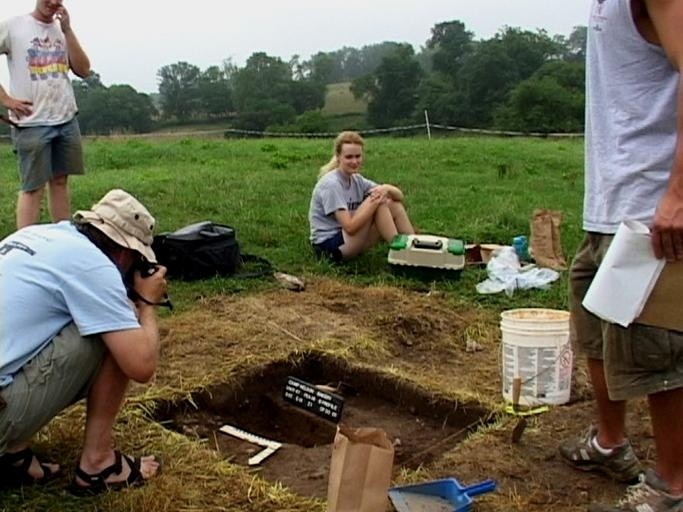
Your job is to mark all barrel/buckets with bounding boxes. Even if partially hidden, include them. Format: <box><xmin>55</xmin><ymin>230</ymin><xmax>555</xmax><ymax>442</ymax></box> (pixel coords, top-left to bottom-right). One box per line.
<box><xmin>499</xmin><ymin>308</ymin><xmax>573</xmax><ymax>404</ymax></box>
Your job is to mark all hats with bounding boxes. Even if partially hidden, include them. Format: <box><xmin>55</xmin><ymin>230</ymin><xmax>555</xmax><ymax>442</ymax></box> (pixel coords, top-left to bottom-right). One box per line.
<box><xmin>71</xmin><ymin>188</ymin><xmax>158</xmax><ymax>264</ymax></box>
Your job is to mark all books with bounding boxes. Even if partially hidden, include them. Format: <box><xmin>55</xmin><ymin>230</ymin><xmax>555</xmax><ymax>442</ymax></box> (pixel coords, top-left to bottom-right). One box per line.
<box><xmin>580</xmin><ymin>218</ymin><xmax>683</xmax><ymax>335</ymax></box>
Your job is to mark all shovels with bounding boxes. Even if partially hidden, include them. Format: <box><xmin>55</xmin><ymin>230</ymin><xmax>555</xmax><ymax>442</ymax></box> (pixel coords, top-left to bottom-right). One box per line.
<box><xmin>384</xmin><ymin>474</ymin><xmax>498</xmax><ymax>512</ymax></box>
<box><xmin>509</xmin><ymin>377</ymin><xmax>527</xmax><ymax>443</ymax></box>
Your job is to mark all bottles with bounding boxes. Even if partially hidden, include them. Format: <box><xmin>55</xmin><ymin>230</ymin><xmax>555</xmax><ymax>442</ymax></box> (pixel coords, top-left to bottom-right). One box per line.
<box><xmin>511</xmin><ymin>235</ymin><xmax>528</xmax><ymax>262</ymax></box>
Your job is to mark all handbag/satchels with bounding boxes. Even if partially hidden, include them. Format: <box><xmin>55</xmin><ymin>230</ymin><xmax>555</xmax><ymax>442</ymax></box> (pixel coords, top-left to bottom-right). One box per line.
<box><xmin>153</xmin><ymin>220</ymin><xmax>239</xmax><ymax>282</ymax></box>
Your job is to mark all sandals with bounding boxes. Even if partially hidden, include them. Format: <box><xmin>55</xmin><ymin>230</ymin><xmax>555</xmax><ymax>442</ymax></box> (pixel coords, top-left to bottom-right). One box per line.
<box><xmin>69</xmin><ymin>450</ymin><xmax>162</xmax><ymax>497</ymax></box>
<box><xmin>0</xmin><ymin>448</ymin><xmax>63</xmax><ymax>488</ymax></box>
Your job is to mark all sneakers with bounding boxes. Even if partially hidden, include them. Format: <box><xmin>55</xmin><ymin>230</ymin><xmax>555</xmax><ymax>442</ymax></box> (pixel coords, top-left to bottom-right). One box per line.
<box><xmin>557</xmin><ymin>426</ymin><xmax>642</xmax><ymax>483</ymax></box>
<box><xmin>587</xmin><ymin>468</ymin><xmax>682</xmax><ymax>512</ymax></box>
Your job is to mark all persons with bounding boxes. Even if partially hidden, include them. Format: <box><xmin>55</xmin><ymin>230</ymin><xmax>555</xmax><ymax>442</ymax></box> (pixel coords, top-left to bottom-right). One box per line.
<box><xmin>0</xmin><ymin>0</ymin><xmax>90</xmax><ymax>231</ymax></box>
<box><xmin>558</xmin><ymin>1</ymin><xmax>683</xmax><ymax>511</ymax></box>
<box><xmin>0</xmin><ymin>186</ymin><xmax>167</xmax><ymax>497</ymax></box>
<box><xmin>308</xmin><ymin>131</ymin><xmax>415</xmax><ymax>265</ymax></box>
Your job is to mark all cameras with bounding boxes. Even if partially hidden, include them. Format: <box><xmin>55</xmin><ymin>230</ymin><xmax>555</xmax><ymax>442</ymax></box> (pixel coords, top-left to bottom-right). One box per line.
<box><xmin>122</xmin><ymin>262</ymin><xmax>163</xmax><ymax>301</ymax></box>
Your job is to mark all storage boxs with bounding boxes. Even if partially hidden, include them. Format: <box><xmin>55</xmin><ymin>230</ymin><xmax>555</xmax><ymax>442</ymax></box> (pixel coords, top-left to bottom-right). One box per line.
<box><xmin>385</xmin><ymin>232</ymin><xmax>466</xmax><ymax>280</ymax></box>
